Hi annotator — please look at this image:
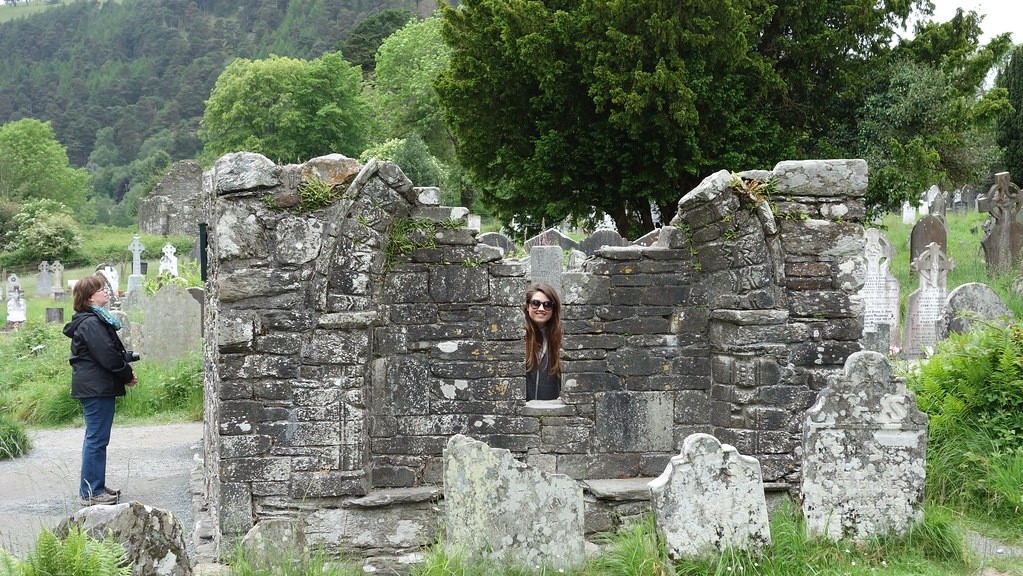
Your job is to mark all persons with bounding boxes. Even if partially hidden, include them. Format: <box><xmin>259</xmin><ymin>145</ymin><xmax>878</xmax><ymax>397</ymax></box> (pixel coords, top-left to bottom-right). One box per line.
<box><xmin>523</xmin><ymin>282</ymin><xmax>561</xmax><ymax>401</ymax></box>
<box><xmin>63</xmin><ymin>277</ymin><xmax>137</xmax><ymax>505</ymax></box>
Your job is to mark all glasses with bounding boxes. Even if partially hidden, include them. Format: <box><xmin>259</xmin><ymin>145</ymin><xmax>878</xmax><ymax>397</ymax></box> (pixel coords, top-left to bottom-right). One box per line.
<box><xmin>530</xmin><ymin>300</ymin><xmax>555</xmax><ymax>311</ymax></box>
<box><xmin>96</xmin><ymin>288</ymin><xmax>108</xmax><ymax>292</ymax></box>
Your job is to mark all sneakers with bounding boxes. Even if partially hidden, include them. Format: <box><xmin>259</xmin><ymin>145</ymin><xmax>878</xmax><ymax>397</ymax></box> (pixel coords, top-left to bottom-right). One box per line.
<box><xmin>81</xmin><ymin>486</ymin><xmax>121</xmax><ymax>505</ymax></box>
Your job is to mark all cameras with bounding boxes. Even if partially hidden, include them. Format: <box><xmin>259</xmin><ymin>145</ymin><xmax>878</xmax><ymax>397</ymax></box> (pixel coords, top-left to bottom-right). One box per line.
<box><xmin>124</xmin><ymin>351</ymin><xmax>140</xmax><ymax>362</ymax></box>
